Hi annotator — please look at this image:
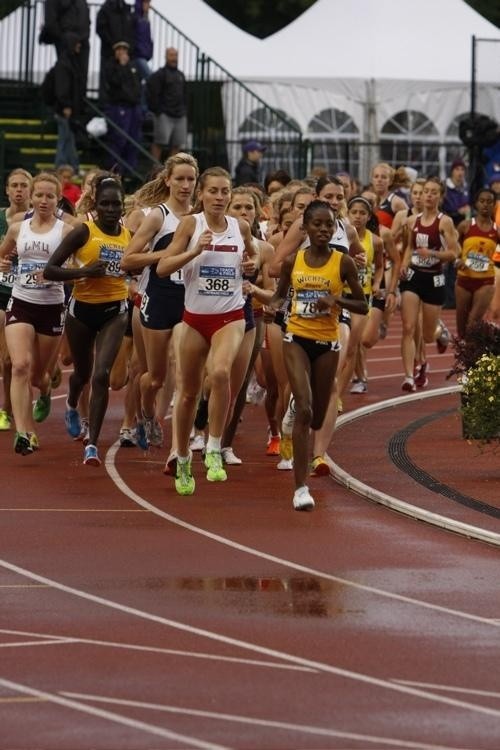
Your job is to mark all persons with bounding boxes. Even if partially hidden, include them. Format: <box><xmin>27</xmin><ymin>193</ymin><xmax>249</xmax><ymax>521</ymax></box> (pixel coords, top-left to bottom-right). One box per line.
<box><xmin>40</xmin><ymin>1</ymin><xmax>192</xmax><ymax>141</ymax></box>
<box><xmin>2</xmin><ymin>138</ymin><xmax>500</xmax><ymax>511</ymax></box>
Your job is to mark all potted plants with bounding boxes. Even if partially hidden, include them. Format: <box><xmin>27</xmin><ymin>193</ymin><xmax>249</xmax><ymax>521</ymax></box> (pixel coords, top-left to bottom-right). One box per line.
<box><xmin>445</xmin><ymin>319</ymin><xmax>500</xmax><ymax>439</ymax></box>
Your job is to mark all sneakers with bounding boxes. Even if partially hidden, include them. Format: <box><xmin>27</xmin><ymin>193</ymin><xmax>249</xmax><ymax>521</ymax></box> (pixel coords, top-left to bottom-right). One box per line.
<box><xmin>0</xmin><ymin>362</ymin><xmax>61</xmax><ymax>455</ymax></box>
<box><xmin>120</xmin><ymin>407</ymin><xmax>164</xmax><ymax>449</ymax></box>
<box><xmin>400</xmin><ymin>360</ymin><xmax>429</xmax><ymax>393</ymax></box>
<box><xmin>162</xmin><ymin>426</ymin><xmax>243</xmax><ymax>496</ymax></box>
<box><xmin>266</xmin><ymin>392</ymin><xmax>331</xmax><ymax>477</ymax></box>
<box><xmin>292</xmin><ymin>486</ymin><xmax>315</xmax><ymax>511</ymax></box>
<box><xmin>64</xmin><ymin>410</ymin><xmax>102</xmax><ymax>467</ymax></box>
<box><xmin>336</xmin><ymin>397</ymin><xmax>344</xmax><ymax>415</ymax></box>
<box><xmin>436</xmin><ymin>319</ymin><xmax>450</xmax><ymax>354</ymax></box>
<box><xmin>245</xmin><ymin>370</ymin><xmax>267</xmax><ymax>407</ymax></box>
<box><xmin>349</xmin><ymin>376</ymin><xmax>368</xmax><ymax>393</ymax></box>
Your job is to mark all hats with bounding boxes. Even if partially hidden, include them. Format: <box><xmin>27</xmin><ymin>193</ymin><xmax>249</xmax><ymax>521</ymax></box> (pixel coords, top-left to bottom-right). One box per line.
<box><xmin>242</xmin><ymin>141</ymin><xmax>268</xmax><ymax>153</ymax></box>
